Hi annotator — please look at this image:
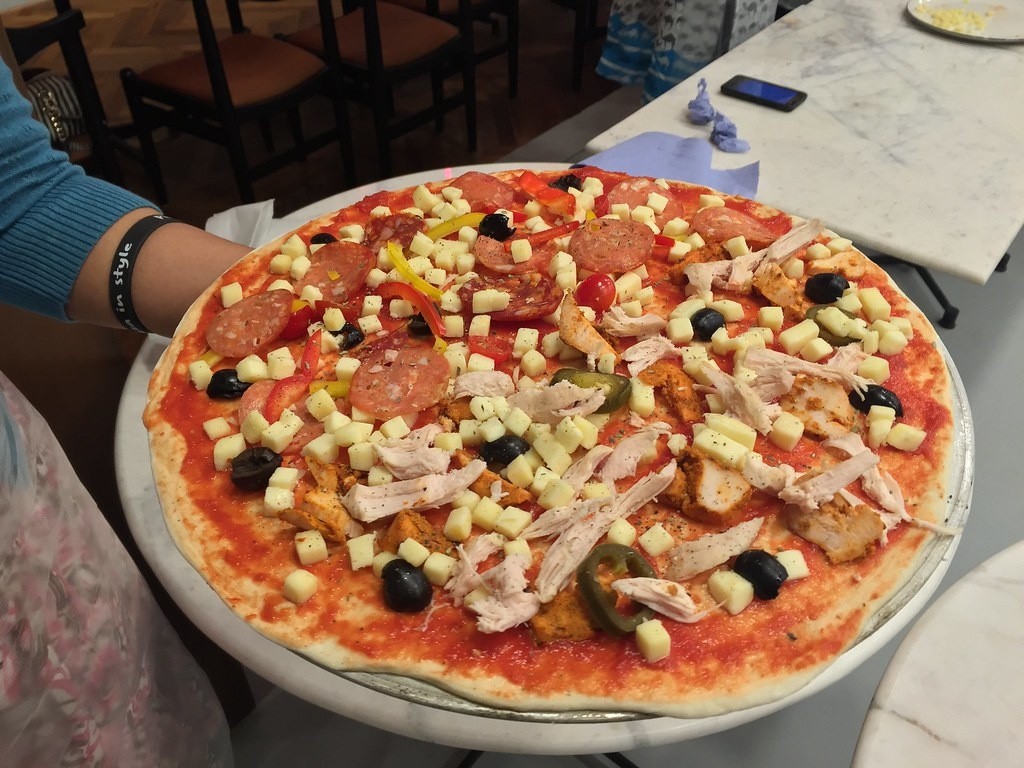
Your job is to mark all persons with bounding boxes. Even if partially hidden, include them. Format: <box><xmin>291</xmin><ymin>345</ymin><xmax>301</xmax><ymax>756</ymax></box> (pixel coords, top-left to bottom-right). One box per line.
<box><xmin>0</xmin><ymin>59</ymin><xmax>257</xmax><ymax>768</ymax></box>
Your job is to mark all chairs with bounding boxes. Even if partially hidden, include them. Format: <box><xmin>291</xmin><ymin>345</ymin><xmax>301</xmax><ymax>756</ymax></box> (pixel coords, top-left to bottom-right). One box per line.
<box><xmin>120</xmin><ymin>0</ymin><xmax>519</xmax><ymax>212</ymax></box>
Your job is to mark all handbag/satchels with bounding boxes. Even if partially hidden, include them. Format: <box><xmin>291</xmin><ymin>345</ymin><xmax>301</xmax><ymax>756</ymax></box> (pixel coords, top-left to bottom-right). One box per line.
<box><xmin>27</xmin><ymin>75</ymin><xmax>86</xmax><ymax>146</ymax></box>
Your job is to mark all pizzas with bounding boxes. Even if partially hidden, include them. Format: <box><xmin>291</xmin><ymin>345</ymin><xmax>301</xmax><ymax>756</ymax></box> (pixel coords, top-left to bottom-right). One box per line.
<box><xmin>141</xmin><ymin>165</ymin><xmax>968</xmax><ymax>720</ymax></box>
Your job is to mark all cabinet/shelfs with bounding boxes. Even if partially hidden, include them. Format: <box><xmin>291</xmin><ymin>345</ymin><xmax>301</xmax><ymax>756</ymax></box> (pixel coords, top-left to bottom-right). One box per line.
<box><xmin>1</xmin><ymin>0</ymin><xmax>126</xmax><ymax>191</ymax></box>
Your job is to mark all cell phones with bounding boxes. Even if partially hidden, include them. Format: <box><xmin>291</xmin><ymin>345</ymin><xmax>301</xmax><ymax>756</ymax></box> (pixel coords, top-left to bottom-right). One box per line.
<box><xmin>722</xmin><ymin>75</ymin><xmax>808</xmax><ymax>112</ymax></box>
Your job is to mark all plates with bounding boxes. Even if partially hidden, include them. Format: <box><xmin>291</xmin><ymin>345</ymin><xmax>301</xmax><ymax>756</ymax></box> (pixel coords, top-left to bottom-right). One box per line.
<box><xmin>906</xmin><ymin>0</ymin><xmax>1024</xmax><ymax>43</ymax></box>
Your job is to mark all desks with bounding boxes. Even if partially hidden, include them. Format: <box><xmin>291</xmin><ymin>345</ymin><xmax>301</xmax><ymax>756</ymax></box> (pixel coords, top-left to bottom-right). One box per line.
<box><xmin>582</xmin><ymin>0</ymin><xmax>1024</xmax><ymax>325</ymax></box>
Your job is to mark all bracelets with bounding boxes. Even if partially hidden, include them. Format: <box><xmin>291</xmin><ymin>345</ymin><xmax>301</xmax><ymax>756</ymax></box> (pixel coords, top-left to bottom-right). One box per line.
<box><xmin>109</xmin><ymin>215</ymin><xmax>184</xmax><ymax>335</ymax></box>
